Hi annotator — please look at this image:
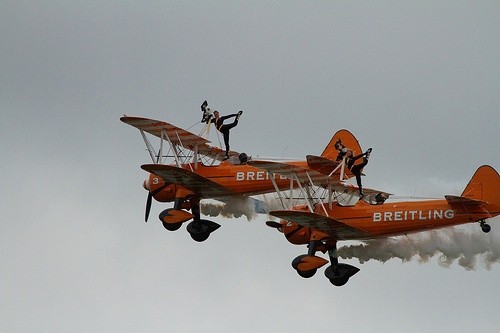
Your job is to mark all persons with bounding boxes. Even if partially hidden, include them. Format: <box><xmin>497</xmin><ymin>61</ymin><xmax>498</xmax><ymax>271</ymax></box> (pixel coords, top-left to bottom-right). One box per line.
<box><xmin>375</xmin><ymin>192</ymin><xmax>389</xmax><ymax>204</ymax></box>
<box><xmin>342</xmin><ymin>147</ymin><xmax>373</xmax><ymax>200</ymax></box>
<box><xmin>238</xmin><ymin>153</ymin><xmax>247</xmax><ymax>165</ymax></box>
<box><xmin>206</xmin><ymin>110</ymin><xmax>243</xmax><ymax>162</ymax></box>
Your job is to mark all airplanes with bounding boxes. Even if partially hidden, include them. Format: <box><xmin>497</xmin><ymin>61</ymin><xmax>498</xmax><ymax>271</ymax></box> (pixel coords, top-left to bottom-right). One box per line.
<box><xmin>120</xmin><ymin>114</ymin><xmax>500</xmax><ymax>287</ymax></box>
